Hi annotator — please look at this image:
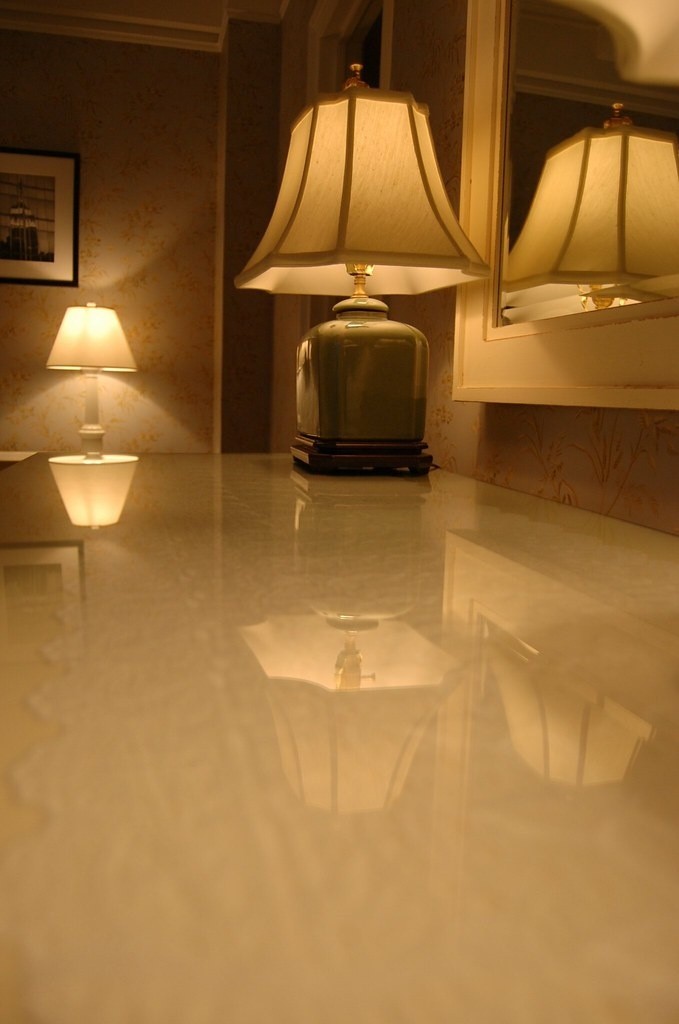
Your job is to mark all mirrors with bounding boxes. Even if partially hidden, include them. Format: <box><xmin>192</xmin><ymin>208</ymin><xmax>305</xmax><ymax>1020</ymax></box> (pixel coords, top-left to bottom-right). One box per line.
<box><xmin>452</xmin><ymin>1</ymin><xmax>679</xmax><ymax>413</ymax></box>
<box><xmin>430</xmin><ymin>528</ymin><xmax>679</xmax><ymax>864</ymax></box>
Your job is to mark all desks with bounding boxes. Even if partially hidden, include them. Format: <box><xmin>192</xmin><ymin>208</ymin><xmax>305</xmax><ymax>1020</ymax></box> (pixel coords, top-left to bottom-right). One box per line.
<box><xmin>0</xmin><ymin>454</ymin><xmax>678</xmax><ymax>1024</ymax></box>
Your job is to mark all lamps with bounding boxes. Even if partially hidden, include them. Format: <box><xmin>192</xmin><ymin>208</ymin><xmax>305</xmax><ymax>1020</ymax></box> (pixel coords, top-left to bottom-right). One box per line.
<box><xmin>493</xmin><ymin>653</ymin><xmax>642</xmax><ymax>789</ymax></box>
<box><xmin>48</xmin><ymin>452</ymin><xmax>139</xmax><ymax>530</ymax></box>
<box><xmin>46</xmin><ymin>302</ymin><xmax>137</xmax><ymax>451</ymax></box>
<box><xmin>500</xmin><ymin>102</ymin><xmax>679</xmax><ymax>323</ymax></box>
<box><xmin>235</xmin><ymin>62</ymin><xmax>489</xmax><ymax>475</ymax></box>
<box><xmin>239</xmin><ymin>471</ymin><xmax>457</xmax><ymax>816</ymax></box>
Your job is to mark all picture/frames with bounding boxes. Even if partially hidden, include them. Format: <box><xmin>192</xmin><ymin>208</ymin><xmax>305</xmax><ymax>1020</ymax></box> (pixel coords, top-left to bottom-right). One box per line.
<box><xmin>0</xmin><ymin>539</ymin><xmax>87</xmax><ymax>664</ymax></box>
<box><xmin>0</xmin><ymin>146</ymin><xmax>80</xmax><ymax>288</ymax></box>
